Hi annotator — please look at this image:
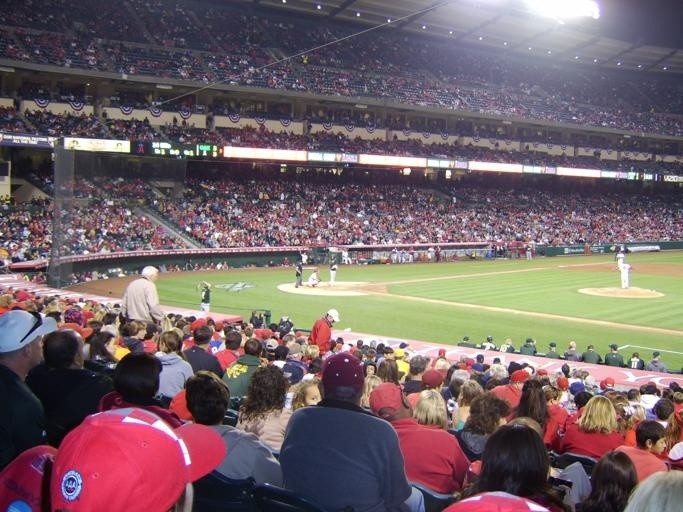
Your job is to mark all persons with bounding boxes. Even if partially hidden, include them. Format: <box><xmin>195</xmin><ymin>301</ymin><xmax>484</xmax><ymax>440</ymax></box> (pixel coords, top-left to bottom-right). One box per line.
<box><xmin>328</xmin><ymin>254</ymin><xmax>338</xmax><ymax>286</ymax></box>
<box><xmin>295</xmin><ymin>260</ymin><xmax>304</xmax><ymax>288</ymax></box>
<box><xmin>22</xmin><ymin>271</ymin><xmax>46</xmax><ymax>283</ymax></box>
<box><xmin>1</xmin><ymin>287</ymin><xmax>683</xmax><ymax>512</ymax></box>
<box><xmin>62</xmin><ymin>271</ymin><xmax>104</xmax><ymax>286</ymax></box>
<box><xmin>0</xmin><ymin>0</ymin><xmax>683</xmax><ymax>273</ymax></box>
<box><xmin>200</xmin><ymin>282</ymin><xmax>210</xmax><ymax>311</ymax></box>
<box><xmin>308</xmin><ymin>267</ymin><xmax>322</xmax><ymax>287</ymax></box>
<box><xmin>619</xmin><ymin>264</ymin><xmax>631</xmax><ymax>288</ymax></box>
<box><xmin>121</xmin><ymin>266</ymin><xmax>167</xmax><ymax>323</ymax></box>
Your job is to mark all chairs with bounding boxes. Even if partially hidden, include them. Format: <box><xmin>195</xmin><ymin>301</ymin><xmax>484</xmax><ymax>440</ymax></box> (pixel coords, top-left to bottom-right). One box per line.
<box><xmin>189</xmin><ymin>469</ymin><xmax>255</xmax><ymax>512</ymax></box>
<box><xmin>408</xmin><ymin>482</ymin><xmax>459</xmax><ymax>512</ymax></box>
<box><xmin>81</xmin><ymin>356</ymin><xmax>244</xmax><ymax>427</ymax></box>
<box><xmin>553</xmin><ymin>452</ymin><xmax>597</xmax><ymax>474</ymax></box>
<box><xmin>254</xmin><ymin>482</ymin><xmax>327</xmax><ymax>511</ymax></box>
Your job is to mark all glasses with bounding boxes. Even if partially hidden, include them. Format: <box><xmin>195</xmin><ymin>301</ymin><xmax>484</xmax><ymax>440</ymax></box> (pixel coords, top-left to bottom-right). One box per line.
<box><xmin>11</xmin><ymin>306</ymin><xmax>42</xmax><ymax>342</ymax></box>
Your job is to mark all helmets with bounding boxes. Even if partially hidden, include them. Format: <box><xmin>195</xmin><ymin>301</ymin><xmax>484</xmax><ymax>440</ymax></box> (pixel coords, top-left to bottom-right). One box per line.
<box><xmin>487</xmin><ymin>336</ymin><xmax>492</xmax><ymax>342</ymax></box>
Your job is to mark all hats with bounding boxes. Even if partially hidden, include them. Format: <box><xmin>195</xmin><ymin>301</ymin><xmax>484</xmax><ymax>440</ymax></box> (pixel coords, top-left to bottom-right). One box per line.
<box><xmin>0</xmin><ymin>309</ymin><xmax>57</xmax><ymax>353</ymax></box>
<box><xmin>64</xmin><ymin>309</ymin><xmax>83</xmax><ymax>326</ymax></box>
<box><xmin>191</xmin><ymin>309</ymin><xmax>341</xmax><ymax>385</ymax></box>
<box><xmin>50</xmin><ymin>406</ymin><xmax>228</xmax><ymax>512</ymax></box>
<box><xmin>369</xmin><ymin>382</ymin><xmax>404</xmax><ymax>418</ymax></box>
<box><xmin>394</xmin><ymin>338</ymin><xmax>661</xmax><ymax>395</ymax></box>
<box><xmin>321</xmin><ymin>352</ymin><xmax>364</xmax><ymax>399</ymax></box>
